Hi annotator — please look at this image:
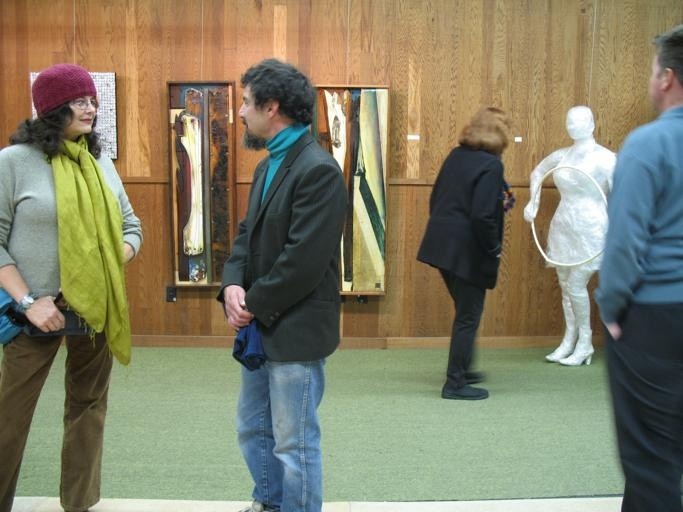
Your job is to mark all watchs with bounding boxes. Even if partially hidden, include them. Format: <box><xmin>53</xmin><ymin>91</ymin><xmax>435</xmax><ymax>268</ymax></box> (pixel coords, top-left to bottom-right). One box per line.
<box><xmin>13</xmin><ymin>291</ymin><xmax>40</xmax><ymax>316</ymax></box>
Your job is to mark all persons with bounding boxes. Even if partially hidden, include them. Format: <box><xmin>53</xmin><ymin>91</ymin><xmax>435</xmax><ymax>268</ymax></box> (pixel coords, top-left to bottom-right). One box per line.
<box><xmin>0</xmin><ymin>61</ymin><xmax>146</xmax><ymax>512</ymax></box>
<box><xmin>418</xmin><ymin>105</ymin><xmax>516</xmax><ymax>402</ymax></box>
<box><xmin>594</xmin><ymin>23</ymin><xmax>683</xmax><ymax>512</ymax></box>
<box><xmin>523</xmin><ymin>103</ymin><xmax>619</xmax><ymax>368</ymax></box>
<box><xmin>213</xmin><ymin>58</ymin><xmax>352</xmax><ymax>512</ymax></box>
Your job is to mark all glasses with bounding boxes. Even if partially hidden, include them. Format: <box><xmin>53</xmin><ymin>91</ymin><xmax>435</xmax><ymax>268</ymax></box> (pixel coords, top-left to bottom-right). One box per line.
<box><xmin>72</xmin><ymin>98</ymin><xmax>99</xmax><ymax>109</ymax></box>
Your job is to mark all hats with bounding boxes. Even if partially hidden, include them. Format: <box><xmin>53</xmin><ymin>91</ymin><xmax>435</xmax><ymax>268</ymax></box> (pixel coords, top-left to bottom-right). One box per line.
<box><xmin>31</xmin><ymin>62</ymin><xmax>97</xmax><ymax>116</ymax></box>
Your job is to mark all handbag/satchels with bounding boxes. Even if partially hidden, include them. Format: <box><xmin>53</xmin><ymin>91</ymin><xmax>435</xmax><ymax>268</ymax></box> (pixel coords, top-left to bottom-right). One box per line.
<box><xmin>28</xmin><ymin>310</ymin><xmax>109</xmax><ymax>339</ymax></box>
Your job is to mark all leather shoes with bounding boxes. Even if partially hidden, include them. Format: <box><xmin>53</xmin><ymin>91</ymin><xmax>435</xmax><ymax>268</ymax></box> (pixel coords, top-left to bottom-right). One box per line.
<box><xmin>441</xmin><ymin>382</ymin><xmax>489</xmax><ymax>400</ymax></box>
<box><xmin>462</xmin><ymin>369</ymin><xmax>486</xmax><ymax>384</ymax></box>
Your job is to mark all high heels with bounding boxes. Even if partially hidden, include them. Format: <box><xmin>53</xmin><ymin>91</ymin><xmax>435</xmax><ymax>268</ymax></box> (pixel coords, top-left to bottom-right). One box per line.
<box><xmin>560</xmin><ymin>329</ymin><xmax>595</xmax><ymax>366</ymax></box>
<box><xmin>545</xmin><ymin>329</ymin><xmax>574</xmax><ymax>362</ymax></box>
